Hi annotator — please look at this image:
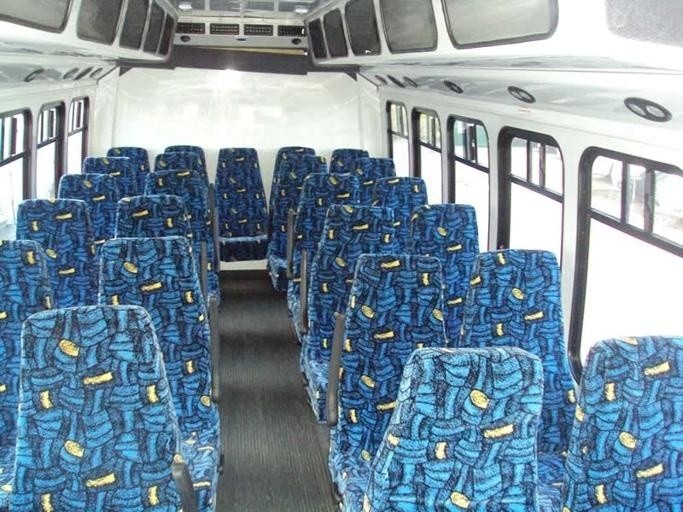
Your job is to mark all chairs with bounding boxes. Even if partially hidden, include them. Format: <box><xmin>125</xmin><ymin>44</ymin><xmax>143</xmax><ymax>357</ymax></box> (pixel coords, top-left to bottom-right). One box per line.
<box><xmin>212</xmin><ymin>148</ymin><xmax>269</xmax><ymax>262</ymax></box>
<box><xmin>1</xmin><ymin>239</ymin><xmax>55</xmax><ymax>510</ymax></box>
<box><xmin>458</xmin><ymin>249</ymin><xmax>579</xmax><ymax>512</ymax></box>
<box><xmin>364</xmin><ymin>347</ymin><xmax>544</xmax><ymax>512</ymax></box>
<box><xmin>328</xmin><ymin>253</ymin><xmax>450</xmax><ymax>512</ymax></box>
<box><xmin>8</xmin><ymin>306</ymin><xmax>197</xmax><ymax>512</ymax></box>
<box><xmin>97</xmin><ymin>235</ymin><xmax>223</xmax><ymax>512</ymax></box>
<box><xmin>561</xmin><ymin>336</ymin><xmax>683</xmax><ymax>512</ymax></box>
<box><xmin>270</xmin><ymin>145</ymin><xmax>480</xmax><ymax>425</ymax></box>
<box><xmin>17</xmin><ymin>146</ymin><xmax>221</xmax><ymax>403</ymax></box>
